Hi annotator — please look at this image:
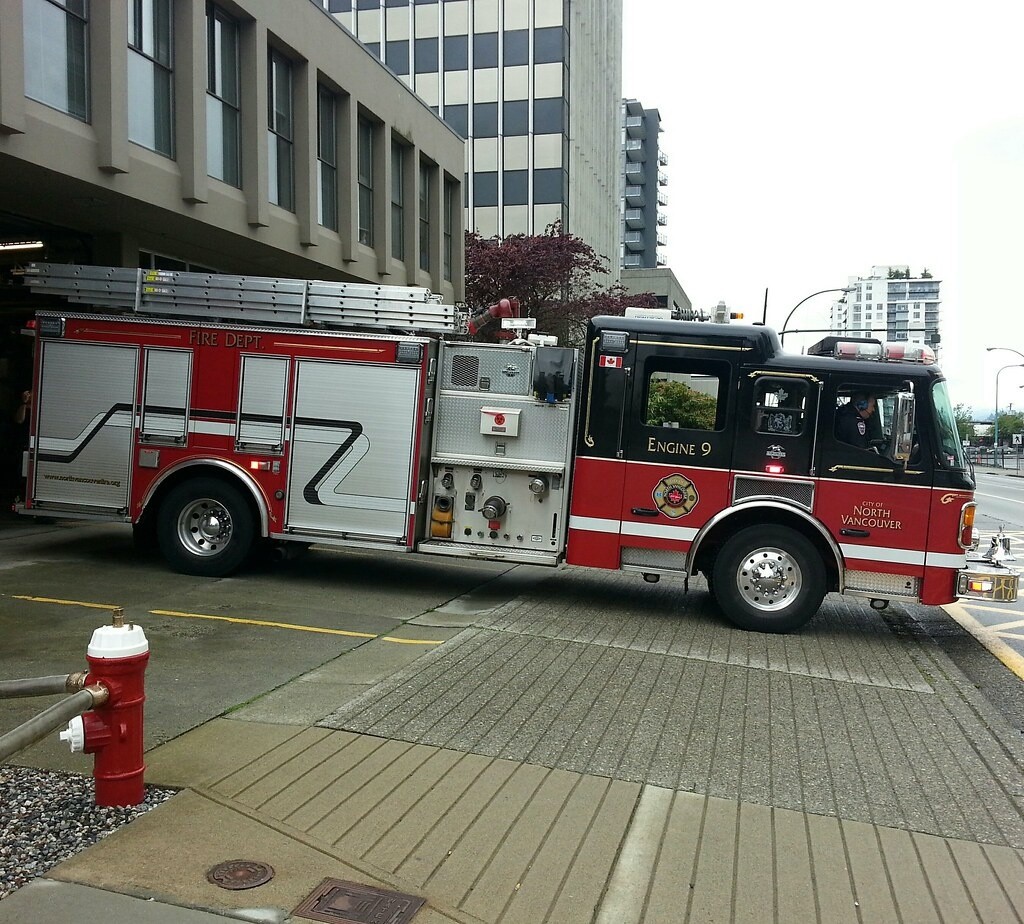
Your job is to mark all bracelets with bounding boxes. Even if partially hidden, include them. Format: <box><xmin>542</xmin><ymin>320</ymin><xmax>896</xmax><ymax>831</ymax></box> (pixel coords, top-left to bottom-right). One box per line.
<box><xmin>20</xmin><ymin>402</ymin><xmax>27</xmax><ymax>405</ymax></box>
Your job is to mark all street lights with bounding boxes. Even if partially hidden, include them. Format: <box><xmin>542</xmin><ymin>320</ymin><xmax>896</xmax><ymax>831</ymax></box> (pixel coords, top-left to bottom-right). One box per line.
<box><xmin>994</xmin><ymin>363</ymin><xmax>1024</xmax><ymax>465</ymax></box>
<box><xmin>778</xmin><ymin>287</ymin><xmax>858</xmax><ymax>354</ymax></box>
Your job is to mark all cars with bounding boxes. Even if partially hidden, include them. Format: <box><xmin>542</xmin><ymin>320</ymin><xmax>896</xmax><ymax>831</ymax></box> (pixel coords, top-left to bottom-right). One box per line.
<box><xmin>962</xmin><ymin>445</ymin><xmax>988</xmax><ymax>455</ymax></box>
<box><xmin>988</xmin><ymin>446</ymin><xmax>1014</xmax><ymax>455</ymax></box>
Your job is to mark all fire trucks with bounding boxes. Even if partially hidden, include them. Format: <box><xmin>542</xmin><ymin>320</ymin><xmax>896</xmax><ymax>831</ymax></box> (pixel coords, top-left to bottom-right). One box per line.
<box><xmin>0</xmin><ymin>239</ymin><xmax>1022</xmax><ymax>634</ymax></box>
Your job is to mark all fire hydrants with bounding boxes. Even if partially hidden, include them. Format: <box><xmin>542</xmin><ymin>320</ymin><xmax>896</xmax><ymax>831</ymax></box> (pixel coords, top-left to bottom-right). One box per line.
<box><xmin>58</xmin><ymin>607</ymin><xmax>152</xmax><ymax>809</ymax></box>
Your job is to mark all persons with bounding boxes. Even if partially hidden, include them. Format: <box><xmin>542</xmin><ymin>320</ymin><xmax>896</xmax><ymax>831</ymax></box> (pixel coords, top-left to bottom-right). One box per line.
<box><xmin>834</xmin><ymin>390</ymin><xmax>876</xmax><ymax>450</ymax></box>
<box><xmin>12</xmin><ymin>380</ymin><xmax>32</xmax><ymax>520</ymax></box>
